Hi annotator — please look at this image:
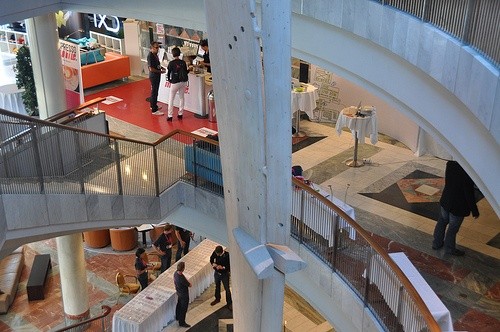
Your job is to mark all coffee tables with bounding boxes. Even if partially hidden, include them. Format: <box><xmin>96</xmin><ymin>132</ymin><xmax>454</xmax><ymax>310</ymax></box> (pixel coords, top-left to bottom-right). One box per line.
<box><xmin>25</xmin><ymin>253</ymin><xmax>52</xmax><ymax>301</ymax></box>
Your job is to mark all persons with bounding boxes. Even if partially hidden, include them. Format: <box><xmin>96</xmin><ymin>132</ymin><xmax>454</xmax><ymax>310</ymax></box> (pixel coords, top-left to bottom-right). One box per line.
<box><xmin>146</xmin><ymin>42</ymin><xmax>166</xmax><ymax>116</ymax></box>
<box><xmin>173</xmin><ymin>262</ymin><xmax>192</xmax><ymax>327</ymax></box>
<box><xmin>200</xmin><ymin>40</ymin><xmax>211</xmax><ymax>73</ymax></box>
<box><xmin>152</xmin><ymin>224</ymin><xmax>193</xmax><ymax>273</ymax></box>
<box><xmin>165</xmin><ymin>47</ymin><xmax>188</xmax><ymax>122</ymax></box>
<box><xmin>210</xmin><ymin>246</ymin><xmax>233</xmax><ymax>306</ymax></box>
<box><xmin>135</xmin><ymin>248</ymin><xmax>149</xmax><ymax>290</ymax></box>
<box><xmin>432</xmin><ymin>161</ymin><xmax>480</xmax><ymax>257</ymax></box>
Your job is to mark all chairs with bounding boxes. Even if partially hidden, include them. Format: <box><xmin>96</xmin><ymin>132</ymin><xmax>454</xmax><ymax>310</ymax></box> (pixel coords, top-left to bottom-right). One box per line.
<box><xmin>144</xmin><ymin>252</ymin><xmax>163</xmax><ymax>278</ymax></box>
<box><xmin>115</xmin><ymin>272</ymin><xmax>141</xmax><ymax>306</ymax></box>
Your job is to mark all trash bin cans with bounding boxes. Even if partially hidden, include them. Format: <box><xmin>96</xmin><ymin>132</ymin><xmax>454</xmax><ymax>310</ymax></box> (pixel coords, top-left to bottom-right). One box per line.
<box><xmin>208</xmin><ymin>91</ymin><xmax>217</xmax><ymax>122</ymax></box>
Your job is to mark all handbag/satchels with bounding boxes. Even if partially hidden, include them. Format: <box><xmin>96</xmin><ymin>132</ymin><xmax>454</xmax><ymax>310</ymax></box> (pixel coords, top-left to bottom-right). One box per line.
<box><xmin>179</xmin><ymin>60</ymin><xmax>188</xmax><ymax>82</ymax></box>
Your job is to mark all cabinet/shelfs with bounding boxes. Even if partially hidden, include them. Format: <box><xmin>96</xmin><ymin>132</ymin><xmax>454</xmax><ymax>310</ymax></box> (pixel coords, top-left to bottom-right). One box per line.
<box><xmin>0</xmin><ymin>30</ymin><xmax>30</xmax><ymax>59</ymax></box>
<box><xmin>156</xmin><ymin>68</ymin><xmax>213</xmax><ymax>117</ymax></box>
<box><xmin>89</xmin><ymin>31</ymin><xmax>127</xmax><ymax>83</ymax></box>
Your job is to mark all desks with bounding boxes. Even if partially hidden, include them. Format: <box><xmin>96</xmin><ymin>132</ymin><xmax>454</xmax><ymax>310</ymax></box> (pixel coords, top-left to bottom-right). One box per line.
<box><xmin>334</xmin><ymin>106</ymin><xmax>378</xmax><ymax>167</ymax></box>
<box><xmin>0</xmin><ymin>84</ymin><xmax>31</xmax><ymax>115</ymax></box>
<box><xmin>291</xmin><ymin>181</ymin><xmax>356</xmax><ymax>248</ymax></box>
<box><xmin>111</xmin><ymin>238</ymin><xmax>227</xmax><ymax>332</ymax></box>
<box><xmin>137</xmin><ymin>225</ymin><xmax>153</xmax><ymax>247</ymax></box>
<box><xmin>291</xmin><ymin>84</ymin><xmax>321</xmax><ymax>139</ymax></box>
<box><xmin>361</xmin><ymin>252</ymin><xmax>455</xmax><ymax>331</ymax></box>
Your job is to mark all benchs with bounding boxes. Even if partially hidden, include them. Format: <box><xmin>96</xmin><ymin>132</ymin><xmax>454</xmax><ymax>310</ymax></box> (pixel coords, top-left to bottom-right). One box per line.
<box><xmin>184</xmin><ymin>135</ymin><xmax>223</xmax><ymax>187</ymax></box>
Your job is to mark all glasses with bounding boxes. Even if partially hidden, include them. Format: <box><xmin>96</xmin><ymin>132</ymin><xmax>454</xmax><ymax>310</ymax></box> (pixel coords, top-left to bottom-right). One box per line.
<box><xmin>154</xmin><ymin>47</ymin><xmax>158</xmax><ymax>49</ymax></box>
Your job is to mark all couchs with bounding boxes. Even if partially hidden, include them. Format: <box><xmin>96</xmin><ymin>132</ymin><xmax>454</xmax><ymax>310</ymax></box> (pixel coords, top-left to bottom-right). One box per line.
<box><xmin>0</xmin><ymin>247</ymin><xmax>25</xmax><ymax>313</ymax></box>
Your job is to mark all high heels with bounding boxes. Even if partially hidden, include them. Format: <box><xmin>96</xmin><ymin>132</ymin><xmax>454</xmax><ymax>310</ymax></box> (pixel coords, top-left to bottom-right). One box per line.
<box><xmin>167</xmin><ymin>117</ymin><xmax>173</xmax><ymax>122</ymax></box>
<box><xmin>177</xmin><ymin>115</ymin><xmax>182</xmax><ymax>119</ymax></box>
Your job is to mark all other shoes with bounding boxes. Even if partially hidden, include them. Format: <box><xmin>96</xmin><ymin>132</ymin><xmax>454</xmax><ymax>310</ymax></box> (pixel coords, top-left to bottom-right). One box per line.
<box><xmin>179</xmin><ymin>323</ymin><xmax>190</xmax><ymax>327</ymax></box>
<box><xmin>211</xmin><ymin>299</ymin><xmax>220</xmax><ymax>306</ymax></box>
<box><xmin>152</xmin><ymin>111</ymin><xmax>164</xmax><ymax>115</ymax></box>
<box><xmin>227</xmin><ymin>303</ymin><xmax>232</xmax><ymax>311</ymax></box>
<box><xmin>150</xmin><ymin>105</ymin><xmax>162</xmax><ymax>109</ymax></box>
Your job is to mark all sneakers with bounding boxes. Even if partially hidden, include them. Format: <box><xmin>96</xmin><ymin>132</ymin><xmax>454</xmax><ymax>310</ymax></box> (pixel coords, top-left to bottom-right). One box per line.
<box><xmin>432</xmin><ymin>243</ymin><xmax>444</xmax><ymax>249</ymax></box>
<box><xmin>452</xmin><ymin>249</ymin><xmax>464</xmax><ymax>256</ymax></box>
